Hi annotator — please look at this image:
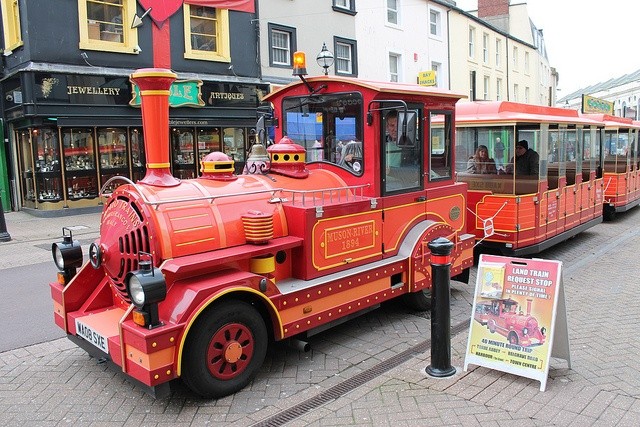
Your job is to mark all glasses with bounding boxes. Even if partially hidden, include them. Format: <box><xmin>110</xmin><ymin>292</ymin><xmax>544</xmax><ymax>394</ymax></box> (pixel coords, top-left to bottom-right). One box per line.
<box><xmin>516</xmin><ymin>147</ymin><xmax>523</xmax><ymax>149</ymax></box>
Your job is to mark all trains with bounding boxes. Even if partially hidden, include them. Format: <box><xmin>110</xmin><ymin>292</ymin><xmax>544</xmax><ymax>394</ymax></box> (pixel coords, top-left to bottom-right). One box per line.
<box><xmin>49</xmin><ymin>50</ymin><xmax>640</xmax><ymax>399</ymax></box>
<box><xmin>474</xmin><ymin>296</ymin><xmax>547</xmax><ymax>346</ymax></box>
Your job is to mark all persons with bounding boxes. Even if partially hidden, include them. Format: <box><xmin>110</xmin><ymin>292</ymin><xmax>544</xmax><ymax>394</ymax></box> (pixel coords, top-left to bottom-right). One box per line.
<box><xmin>498</xmin><ymin>138</ymin><xmax>540</xmax><ymax>176</ymax></box>
<box><xmin>467</xmin><ymin>144</ymin><xmax>497</xmax><ymax>175</ymax></box>
<box><xmin>336</xmin><ymin>139</ymin><xmax>344</xmax><ymax>149</ymax></box>
<box><xmin>493</xmin><ymin>135</ymin><xmax>506</xmax><ymax>169</ymax></box>
<box><xmin>311</xmin><ymin>138</ymin><xmax>325</xmax><ymax>163</ymax></box>
<box><xmin>345</xmin><ymin>108</ymin><xmax>413</xmax><ymax>191</ymax></box>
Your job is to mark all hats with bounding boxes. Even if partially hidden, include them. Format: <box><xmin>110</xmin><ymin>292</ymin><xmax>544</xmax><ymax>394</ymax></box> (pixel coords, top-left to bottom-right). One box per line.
<box><xmin>517</xmin><ymin>140</ymin><xmax>528</xmax><ymax>151</ymax></box>
